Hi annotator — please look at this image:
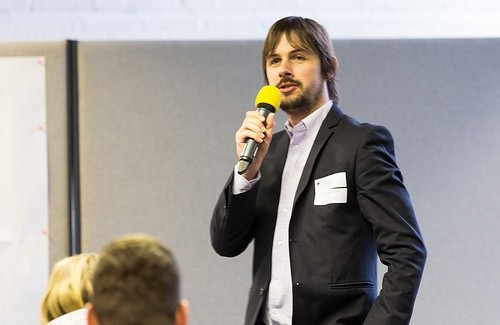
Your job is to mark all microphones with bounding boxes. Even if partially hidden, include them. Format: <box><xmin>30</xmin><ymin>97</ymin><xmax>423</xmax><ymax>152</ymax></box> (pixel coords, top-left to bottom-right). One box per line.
<box><xmin>238</xmin><ymin>85</ymin><xmax>282</xmax><ymax>175</ymax></box>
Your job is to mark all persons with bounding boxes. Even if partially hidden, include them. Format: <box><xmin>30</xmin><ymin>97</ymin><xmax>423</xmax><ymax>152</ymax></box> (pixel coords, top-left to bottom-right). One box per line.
<box><xmin>87</xmin><ymin>236</ymin><xmax>188</xmax><ymax>325</ymax></box>
<box><xmin>41</xmin><ymin>250</ymin><xmax>100</xmax><ymax>325</ymax></box>
<box><xmin>209</xmin><ymin>16</ymin><xmax>427</xmax><ymax>325</ymax></box>
